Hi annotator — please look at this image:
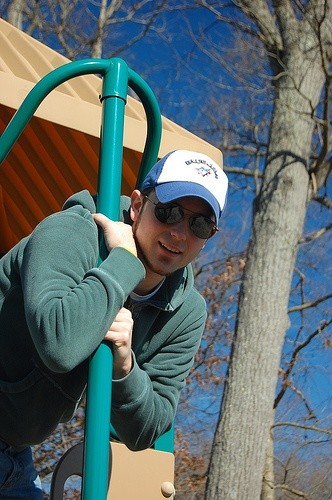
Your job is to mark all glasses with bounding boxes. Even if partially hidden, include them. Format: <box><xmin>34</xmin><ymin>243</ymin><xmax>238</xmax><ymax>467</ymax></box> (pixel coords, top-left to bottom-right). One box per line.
<box><xmin>145</xmin><ymin>195</ymin><xmax>220</xmax><ymax>239</ymax></box>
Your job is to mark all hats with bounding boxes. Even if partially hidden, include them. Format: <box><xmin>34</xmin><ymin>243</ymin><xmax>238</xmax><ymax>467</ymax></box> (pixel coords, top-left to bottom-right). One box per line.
<box><xmin>141</xmin><ymin>148</ymin><xmax>229</xmax><ymax>231</ymax></box>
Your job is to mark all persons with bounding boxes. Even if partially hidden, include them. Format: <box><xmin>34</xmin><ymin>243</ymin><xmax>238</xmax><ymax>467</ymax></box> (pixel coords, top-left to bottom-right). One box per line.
<box><xmin>0</xmin><ymin>151</ymin><xmax>227</xmax><ymax>500</ymax></box>
